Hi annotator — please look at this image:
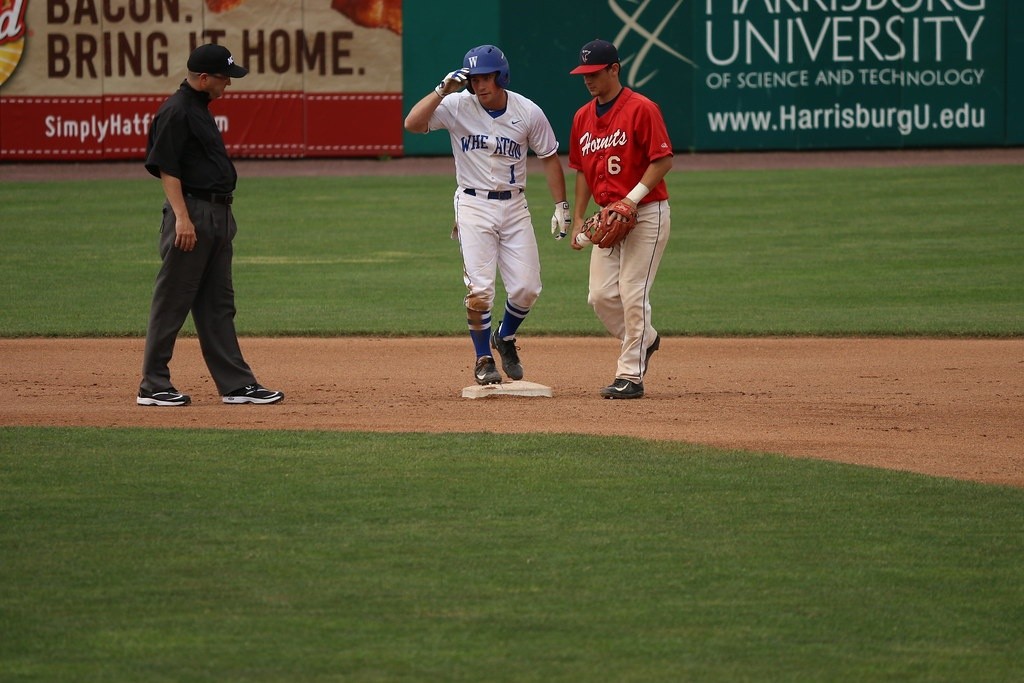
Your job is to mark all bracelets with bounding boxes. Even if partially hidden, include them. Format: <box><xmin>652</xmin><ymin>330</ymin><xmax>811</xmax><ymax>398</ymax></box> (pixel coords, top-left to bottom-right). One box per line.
<box><xmin>626</xmin><ymin>181</ymin><xmax>650</xmax><ymax>204</ymax></box>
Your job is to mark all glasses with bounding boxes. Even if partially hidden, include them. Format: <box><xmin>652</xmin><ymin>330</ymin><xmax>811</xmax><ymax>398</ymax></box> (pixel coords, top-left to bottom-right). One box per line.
<box><xmin>198</xmin><ymin>73</ymin><xmax>230</xmax><ymax>82</ymax></box>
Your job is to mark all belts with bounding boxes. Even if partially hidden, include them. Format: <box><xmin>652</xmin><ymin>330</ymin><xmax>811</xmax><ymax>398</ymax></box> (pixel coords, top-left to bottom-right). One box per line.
<box><xmin>183</xmin><ymin>188</ymin><xmax>233</xmax><ymax>204</ymax></box>
<box><xmin>464</xmin><ymin>189</ymin><xmax>523</xmax><ymax>200</ymax></box>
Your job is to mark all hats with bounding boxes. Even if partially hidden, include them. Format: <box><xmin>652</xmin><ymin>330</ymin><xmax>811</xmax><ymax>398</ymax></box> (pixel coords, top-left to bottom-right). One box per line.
<box><xmin>569</xmin><ymin>39</ymin><xmax>617</xmax><ymax>75</ymax></box>
<box><xmin>187</xmin><ymin>44</ymin><xmax>249</xmax><ymax>78</ymax></box>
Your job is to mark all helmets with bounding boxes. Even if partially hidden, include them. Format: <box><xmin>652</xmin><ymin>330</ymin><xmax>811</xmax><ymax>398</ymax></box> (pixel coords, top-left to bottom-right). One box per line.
<box><xmin>463</xmin><ymin>45</ymin><xmax>510</xmax><ymax>95</ymax></box>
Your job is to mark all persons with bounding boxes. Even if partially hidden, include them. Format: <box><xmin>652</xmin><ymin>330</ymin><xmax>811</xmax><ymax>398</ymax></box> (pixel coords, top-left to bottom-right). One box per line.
<box><xmin>136</xmin><ymin>45</ymin><xmax>285</xmax><ymax>407</ymax></box>
<box><xmin>569</xmin><ymin>39</ymin><xmax>673</xmax><ymax>398</ymax></box>
<box><xmin>404</xmin><ymin>46</ymin><xmax>572</xmax><ymax>385</ymax></box>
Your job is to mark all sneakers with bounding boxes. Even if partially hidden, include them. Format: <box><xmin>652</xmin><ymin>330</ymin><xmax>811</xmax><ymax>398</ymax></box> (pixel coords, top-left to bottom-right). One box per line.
<box><xmin>643</xmin><ymin>335</ymin><xmax>660</xmax><ymax>376</ymax></box>
<box><xmin>491</xmin><ymin>321</ymin><xmax>524</xmax><ymax>380</ymax></box>
<box><xmin>474</xmin><ymin>355</ymin><xmax>502</xmax><ymax>386</ymax></box>
<box><xmin>137</xmin><ymin>390</ymin><xmax>190</xmax><ymax>406</ymax></box>
<box><xmin>602</xmin><ymin>380</ymin><xmax>644</xmax><ymax>398</ymax></box>
<box><xmin>223</xmin><ymin>383</ymin><xmax>283</xmax><ymax>404</ymax></box>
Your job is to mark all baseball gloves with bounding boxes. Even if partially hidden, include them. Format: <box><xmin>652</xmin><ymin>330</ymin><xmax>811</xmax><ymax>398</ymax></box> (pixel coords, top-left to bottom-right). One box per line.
<box><xmin>582</xmin><ymin>200</ymin><xmax>639</xmax><ymax>250</ymax></box>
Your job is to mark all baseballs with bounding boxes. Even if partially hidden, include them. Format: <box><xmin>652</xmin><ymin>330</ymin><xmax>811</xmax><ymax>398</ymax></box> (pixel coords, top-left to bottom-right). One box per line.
<box><xmin>576</xmin><ymin>231</ymin><xmax>590</xmax><ymax>248</ymax></box>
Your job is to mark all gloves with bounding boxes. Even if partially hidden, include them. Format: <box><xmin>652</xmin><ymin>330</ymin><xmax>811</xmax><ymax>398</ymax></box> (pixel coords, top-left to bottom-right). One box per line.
<box><xmin>551</xmin><ymin>201</ymin><xmax>571</xmax><ymax>240</ymax></box>
<box><xmin>435</xmin><ymin>68</ymin><xmax>470</xmax><ymax>98</ymax></box>
